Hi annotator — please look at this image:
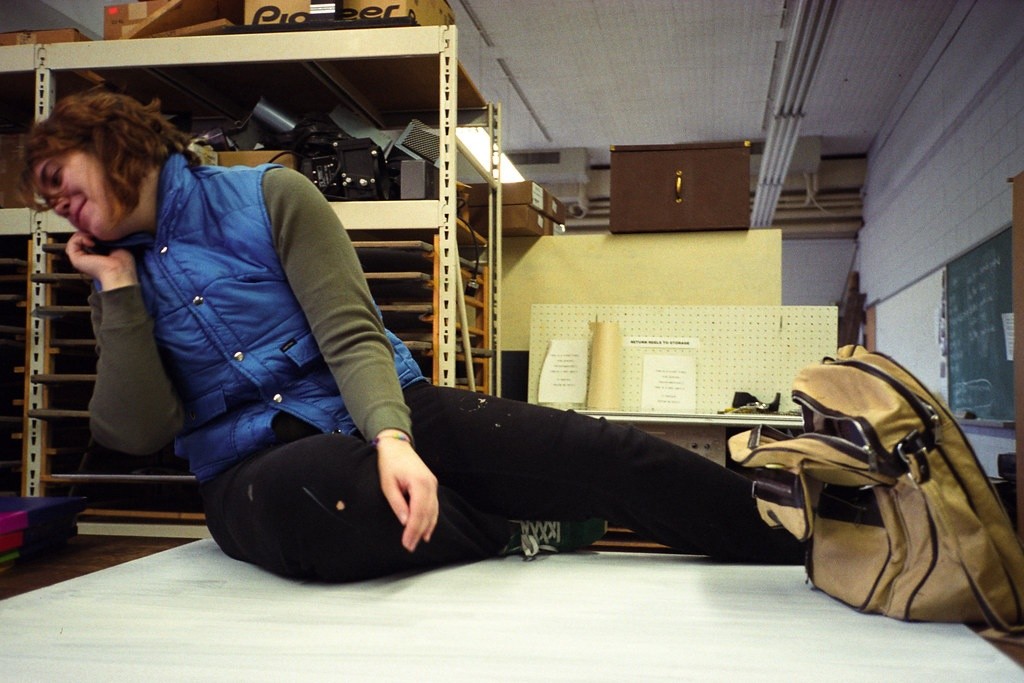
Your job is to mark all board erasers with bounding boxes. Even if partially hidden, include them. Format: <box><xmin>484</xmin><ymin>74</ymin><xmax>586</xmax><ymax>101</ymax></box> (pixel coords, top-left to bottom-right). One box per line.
<box><xmin>955</xmin><ymin>410</ymin><xmax>977</xmax><ymax>419</ymax></box>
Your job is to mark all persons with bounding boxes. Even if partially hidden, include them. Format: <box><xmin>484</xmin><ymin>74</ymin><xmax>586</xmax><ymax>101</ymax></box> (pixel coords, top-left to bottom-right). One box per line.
<box><xmin>15</xmin><ymin>96</ymin><xmax>1019</xmax><ymax>585</ymax></box>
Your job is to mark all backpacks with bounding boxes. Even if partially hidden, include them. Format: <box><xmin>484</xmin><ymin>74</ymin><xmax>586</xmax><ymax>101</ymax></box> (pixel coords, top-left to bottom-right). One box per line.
<box><xmin>728</xmin><ymin>344</ymin><xmax>1024</xmax><ymax>645</ymax></box>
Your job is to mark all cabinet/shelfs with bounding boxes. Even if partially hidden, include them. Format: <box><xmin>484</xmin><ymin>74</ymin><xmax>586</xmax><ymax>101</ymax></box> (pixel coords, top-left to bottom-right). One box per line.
<box><xmin>0</xmin><ymin>25</ymin><xmax>505</xmax><ymax>537</ymax></box>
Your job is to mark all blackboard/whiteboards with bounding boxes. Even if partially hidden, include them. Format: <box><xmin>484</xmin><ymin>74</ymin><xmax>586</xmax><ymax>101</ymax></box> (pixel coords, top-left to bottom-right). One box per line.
<box><xmin>946</xmin><ymin>223</ymin><xmax>1017</xmax><ymax>429</ymax></box>
<box><xmin>875</xmin><ymin>262</ymin><xmax>948</xmax><ymax>408</ymax></box>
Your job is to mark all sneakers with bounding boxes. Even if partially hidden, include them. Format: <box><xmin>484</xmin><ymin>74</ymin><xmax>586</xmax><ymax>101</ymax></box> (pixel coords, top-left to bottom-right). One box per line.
<box><xmin>498</xmin><ymin>517</ymin><xmax>607</xmax><ymax>557</ymax></box>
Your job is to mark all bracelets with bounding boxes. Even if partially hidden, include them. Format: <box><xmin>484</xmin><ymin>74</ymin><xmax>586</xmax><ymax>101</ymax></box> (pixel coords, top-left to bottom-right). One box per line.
<box><xmin>371</xmin><ymin>434</ymin><xmax>411</xmax><ymax>447</ymax></box>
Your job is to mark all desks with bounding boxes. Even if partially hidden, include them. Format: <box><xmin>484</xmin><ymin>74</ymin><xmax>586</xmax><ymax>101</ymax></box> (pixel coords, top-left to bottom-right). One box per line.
<box><xmin>577</xmin><ymin>408</ymin><xmax>804</xmax><ymax>429</ymax></box>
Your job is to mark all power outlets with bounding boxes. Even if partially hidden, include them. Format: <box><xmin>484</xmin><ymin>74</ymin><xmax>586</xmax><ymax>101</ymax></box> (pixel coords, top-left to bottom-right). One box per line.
<box><xmin>686</xmin><ymin>439</ymin><xmax>712</xmax><ymax>454</ymax></box>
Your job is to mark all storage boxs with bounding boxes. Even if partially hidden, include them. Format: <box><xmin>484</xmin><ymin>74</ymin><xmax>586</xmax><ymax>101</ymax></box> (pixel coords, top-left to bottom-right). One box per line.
<box><xmin>400</xmin><ymin>158</ymin><xmax>436</xmax><ymax>200</ymax></box>
<box><xmin>0</xmin><ymin>0</ymin><xmax>456</xmax><ymax>47</ymax></box>
<box><xmin>465</xmin><ymin>179</ymin><xmax>566</xmax><ymax>224</ymax></box>
<box><xmin>469</xmin><ymin>207</ymin><xmax>553</xmax><ymax>236</ymax></box>
<box><xmin>608</xmin><ymin>139</ymin><xmax>753</xmax><ymax>232</ymax></box>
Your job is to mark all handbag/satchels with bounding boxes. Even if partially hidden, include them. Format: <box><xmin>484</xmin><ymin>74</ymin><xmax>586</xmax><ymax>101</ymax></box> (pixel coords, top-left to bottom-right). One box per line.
<box><xmin>270</xmin><ymin>122</ymin><xmax>393</xmax><ymax>200</ymax></box>
